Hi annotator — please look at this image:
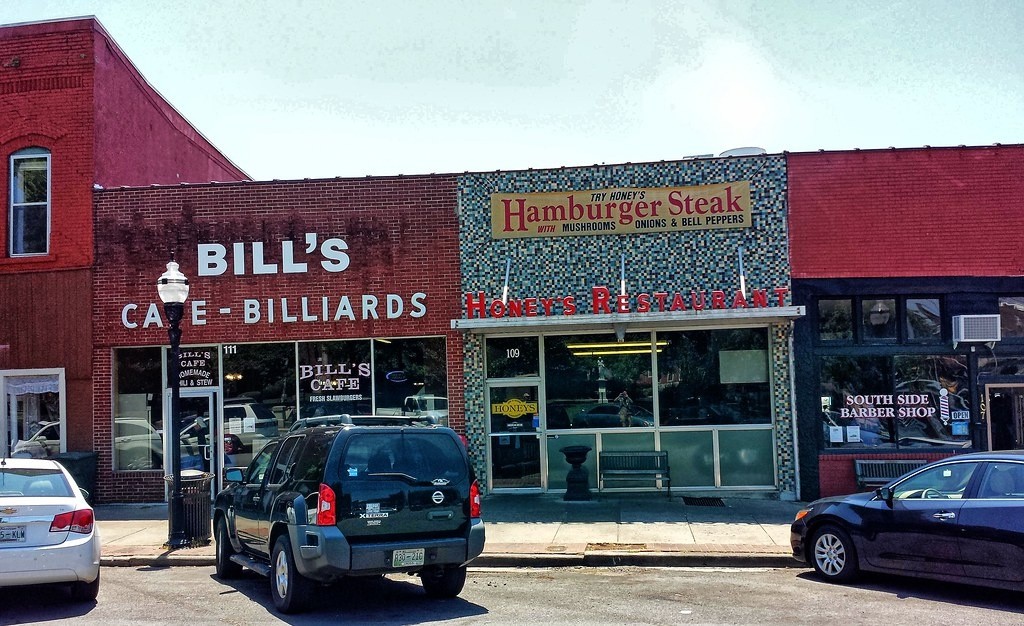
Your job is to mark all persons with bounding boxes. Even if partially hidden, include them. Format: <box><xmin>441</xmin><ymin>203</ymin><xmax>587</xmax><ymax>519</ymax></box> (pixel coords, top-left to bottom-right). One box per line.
<box><xmin>613</xmin><ymin>389</ymin><xmax>634</xmax><ymax>427</ymax></box>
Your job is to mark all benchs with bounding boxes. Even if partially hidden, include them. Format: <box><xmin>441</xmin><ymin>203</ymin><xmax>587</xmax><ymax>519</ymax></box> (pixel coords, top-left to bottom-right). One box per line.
<box><xmin>599</xmin><ymin>450</ymin><xmax>672</xmax><ymax>501</ymax></box>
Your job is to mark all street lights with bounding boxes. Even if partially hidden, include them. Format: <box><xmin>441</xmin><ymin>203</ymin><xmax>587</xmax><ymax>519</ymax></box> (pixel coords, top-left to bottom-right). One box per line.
<box><xmin>153</xmin><ymin>252</ymin><xmax>190</xmax><ymax>549</ymax></box>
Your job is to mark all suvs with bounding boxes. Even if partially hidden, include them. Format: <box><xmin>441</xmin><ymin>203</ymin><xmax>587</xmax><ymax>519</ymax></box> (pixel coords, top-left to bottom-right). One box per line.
<box><xmin>211</xmin><ymin>412</ymin><xmax>487</xmax><ymax>614</ymax></box>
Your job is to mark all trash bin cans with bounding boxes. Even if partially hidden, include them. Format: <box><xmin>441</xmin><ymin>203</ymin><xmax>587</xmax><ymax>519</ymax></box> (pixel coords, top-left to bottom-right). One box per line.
<box><xmin>163</xmin><ymin>470</ymin><xmax>215</xmax><ymax>546</ymax></box>
<box><xmin>42</xmin><ymin>451</ymin><xmax>99</xmax><ymax>508</ymax></box>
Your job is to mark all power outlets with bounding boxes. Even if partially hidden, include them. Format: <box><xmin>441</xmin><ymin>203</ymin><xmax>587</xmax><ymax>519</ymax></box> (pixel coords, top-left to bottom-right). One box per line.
<box><xmin>822</xmin><ymin>405</ymin><xmax>830</xmax><ymax>413</ymax></box>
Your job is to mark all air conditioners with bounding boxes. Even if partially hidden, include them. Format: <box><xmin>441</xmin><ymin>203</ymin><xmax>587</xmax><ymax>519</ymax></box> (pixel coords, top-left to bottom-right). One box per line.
<box><xmin>952</xmin><ymin>314</ymin><xmax>1003</xmax><ymax>343</ymax></box>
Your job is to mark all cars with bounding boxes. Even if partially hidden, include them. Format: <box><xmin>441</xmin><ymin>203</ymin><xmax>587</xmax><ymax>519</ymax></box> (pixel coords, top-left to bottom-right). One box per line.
<box><xmin>565</xmin><ymin>350</ymin><xmax>1024</xmax><ymax>478</ymax></box>
<box><xmin>789</xmin><ymin>449</ymin><xmax>1024</xmax><ymax>593</ymax></box>
<box><xmin>0</xmin><ymin>452</ymin><xmax>102</xmax><ymax>602</ymax></box>
